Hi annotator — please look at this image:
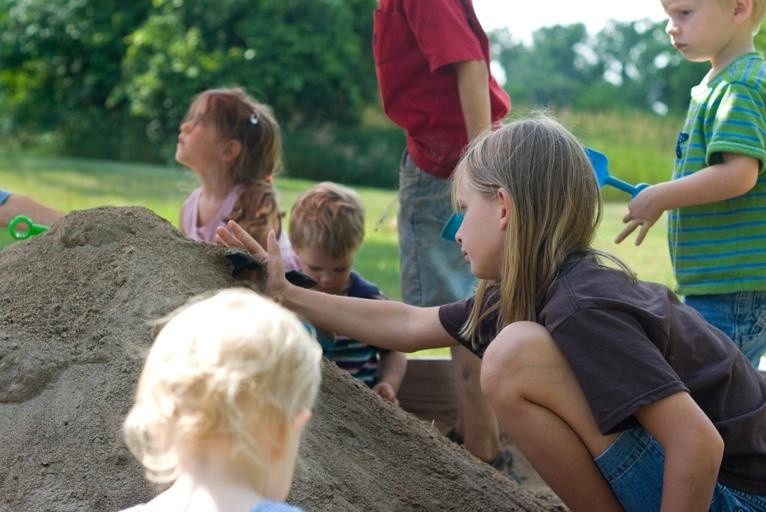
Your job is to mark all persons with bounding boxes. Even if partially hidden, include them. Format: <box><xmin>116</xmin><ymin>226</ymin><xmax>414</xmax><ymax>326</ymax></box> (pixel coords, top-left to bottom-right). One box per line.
<box><xmin>374</xmin><ymin>0</ymin><xmax>511</xmax><ymax>310</ymax></box>
<box><xmin>283</xmin><ymin>181</ymin><xmax>407</xmax><ymax>410</ymax></box>
<box><xmin>116</xmin><ymin>286</ymin><xmax>324</xmax><ymax>512</ymax></box>
<box><xmin>213</xmin><ymin>118</ymin><xmax>766</xmax><ymax>511</ymax></box>
<box><xmin>1</xmin><ymin>189</ymin><xmax>68</xmax><ymax>235</ymax></box>
<box><xmin>615</xmin><ymin>0</ymin><xmax>766</xmax><ymax>374</ymax></box>
<box><xmin>174</xmin><ymin>83</ymin><xmax>311</xmax><ymax>276</ymax></box>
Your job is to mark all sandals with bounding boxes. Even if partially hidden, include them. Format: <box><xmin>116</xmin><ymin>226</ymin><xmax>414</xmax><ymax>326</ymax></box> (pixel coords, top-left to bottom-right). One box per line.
<box><xmin>486</xmin><ymin>450</ymin><xmax>524</xmax><ymax>484</ymax></box>
